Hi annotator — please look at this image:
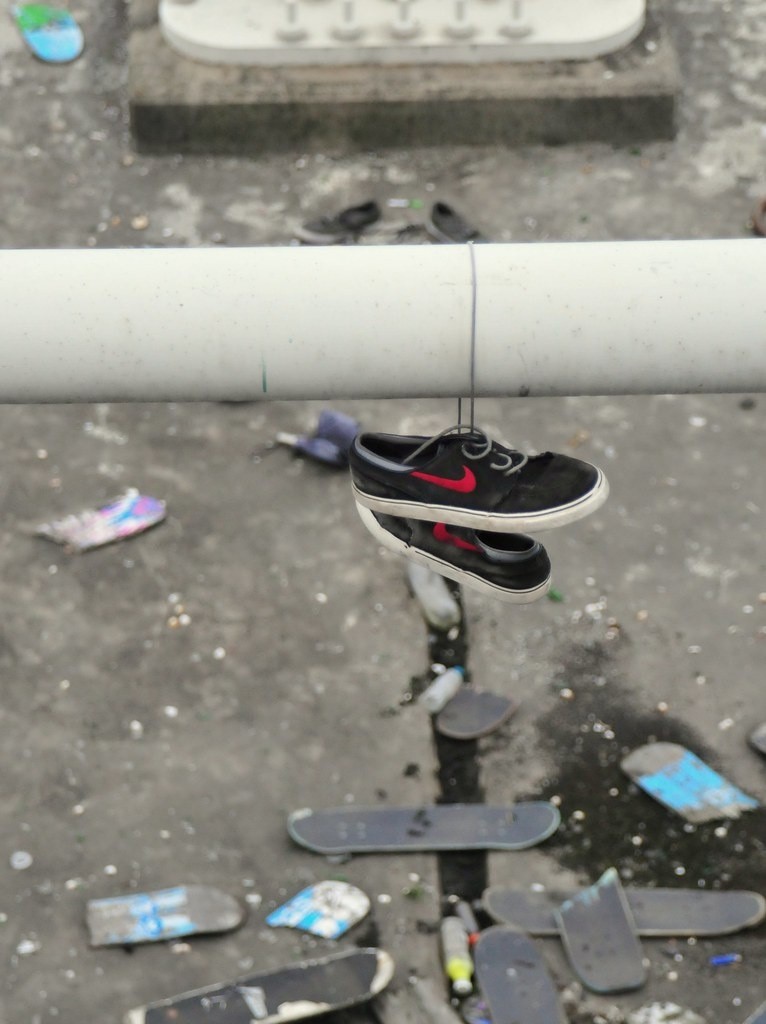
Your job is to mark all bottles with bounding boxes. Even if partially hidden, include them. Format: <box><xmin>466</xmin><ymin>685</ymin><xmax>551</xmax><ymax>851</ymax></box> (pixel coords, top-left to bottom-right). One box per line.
<box><xmin>419</xmin><ymin>665</ymin><xmax>466</xmax><ymax>712</ymax></box>
<box><xmin>441</xmin><ymin>916</ymin><xmax>475</xmax><ymax>993</ymax></box>
<box><xmin>409</xmin><ymin>561</ymin><xmax>461</xmax><ymax>628</ymax></box>
<box><xmin>458</xmin><ymin>901</ymin><xmax>479</xmax><ymax>943</ymax></box>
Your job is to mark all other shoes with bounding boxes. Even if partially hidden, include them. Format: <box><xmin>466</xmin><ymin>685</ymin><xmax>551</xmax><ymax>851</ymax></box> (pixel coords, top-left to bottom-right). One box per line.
<box><xmin>293</xmin><ymin>200</ymin><xmax>382</xmax><ymax>243</ymax></box>
<box><xmin>424</xmin><ymin>202</ymin><xmax>489</xmax><ymax>245</ymax></box>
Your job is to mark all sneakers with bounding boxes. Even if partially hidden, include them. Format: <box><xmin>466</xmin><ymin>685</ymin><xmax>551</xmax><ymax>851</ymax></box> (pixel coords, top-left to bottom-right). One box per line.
<box><xmin>348</xmin><ymin>425</ymin><xmax>610</xmax><ymax>535</ymax></box>
<box><xmin>356</xmin><ymin>499</ymin><xmax>554</xmax><ymax>604</ymax></box>
